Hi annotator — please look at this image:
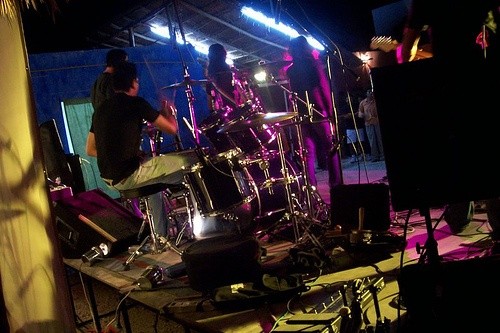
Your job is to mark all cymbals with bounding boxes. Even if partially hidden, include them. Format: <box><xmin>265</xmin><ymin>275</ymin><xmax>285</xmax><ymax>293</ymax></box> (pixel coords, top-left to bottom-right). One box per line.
<box><xmin>218</xmin><ymin>111</ymin><xmax>298</xmax><ymax>133</ymax></box>
<box><xmin>239</xmin><ymin>61</ymin><xmax>293</xmax><ymax>75</ymax></box>
<box><xmin>161</xmin><ymin>78</ymin><xmax>216</xmax><ymax>90</ymax></box>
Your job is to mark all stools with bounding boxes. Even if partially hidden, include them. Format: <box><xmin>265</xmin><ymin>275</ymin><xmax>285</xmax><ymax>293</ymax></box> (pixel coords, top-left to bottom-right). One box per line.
<box><xmin>118</xmin><ymin>182</ymin><xmax>183</xmax><ymax>272</ymax></box>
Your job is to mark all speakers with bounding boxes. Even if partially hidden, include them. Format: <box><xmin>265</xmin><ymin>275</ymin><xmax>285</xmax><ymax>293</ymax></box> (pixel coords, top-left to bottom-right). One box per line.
<box><xmin>371</xmin><ymin>58</ymin><xmax>497</xmax><ymax>212</ymax></box>
<box><xmin>330</xmin><ymin>183</ymin><xmax>390</xmax><ymax>232</ymax></box>
<box><xmin>53</xmin><ymin>188</ymin><xmax>152</xmax><ymax>257</ymax></box>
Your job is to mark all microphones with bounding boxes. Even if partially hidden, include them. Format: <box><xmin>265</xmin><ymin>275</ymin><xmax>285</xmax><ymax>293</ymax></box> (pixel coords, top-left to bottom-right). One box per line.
<box><xmin>275</xmin><ymin>0</ymin><xmax>281</xmax><ymax>25</ymax></box>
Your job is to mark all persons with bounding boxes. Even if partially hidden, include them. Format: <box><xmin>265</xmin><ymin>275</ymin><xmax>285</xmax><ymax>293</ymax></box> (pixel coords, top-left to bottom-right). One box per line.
<box><xmin>90</xmin><ymin>48</ymin><xmax>128</xmax><ymax>109</ymax></box>
<box><xmin>205</xmin><ymin>43</ymin><xmax>233</xmax><ymax>111</ymax></box>
<box><xmin>357</xmin><ymin>89</ymin><xmax>385</xmax><ymax>162</ymax></box>
<box><xmin>85</xmin><ymin>62</ymin><xmax>200</xmax><ymax>241</ymax></box>
<box><xmin>287</xmin><ymin>36</ymin><xmax>343</xmax><ymax>188</ymax></box>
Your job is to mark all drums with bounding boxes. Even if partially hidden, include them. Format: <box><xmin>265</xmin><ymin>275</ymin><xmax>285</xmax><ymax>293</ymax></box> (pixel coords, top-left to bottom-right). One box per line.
<box><xmin>235</xmin><ymin>150</ymin><xmax>304</xmax><ymax>236</ymax></box>
<box><xmin>196</xmin><ymin>106</ymin><xmax>242</xmax><ymax>161</ymax></box>
<box><xmin>215</xmin><ymin>99</ymin><xmax>278</xmax><ymax>159</ymax></box>
<box><xmin>180</xmin><ymin>147</ymin><xmax>257</xmax><ymax>218</ymax></box>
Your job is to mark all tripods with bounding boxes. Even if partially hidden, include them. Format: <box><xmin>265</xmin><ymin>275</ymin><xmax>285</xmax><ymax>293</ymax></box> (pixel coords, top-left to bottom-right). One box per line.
<box><xmin>122</xmin><ymin>192</ymin><xmax>195</xmax><ymax>270</ymax></box>
<box><xmin>257</xmin><ymin>71</ymin><xmax>340</xmax><ymax>263</ymax></box>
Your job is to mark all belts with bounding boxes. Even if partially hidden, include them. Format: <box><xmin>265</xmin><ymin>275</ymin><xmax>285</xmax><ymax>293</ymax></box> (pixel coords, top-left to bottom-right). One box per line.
<box><xmin>103</xmin><ymin>178</ymin><xmax>124</xmax><ymax>187</ymax></box>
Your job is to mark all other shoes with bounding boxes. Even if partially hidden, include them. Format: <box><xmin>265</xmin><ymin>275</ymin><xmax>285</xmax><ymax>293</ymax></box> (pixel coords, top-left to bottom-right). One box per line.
<box><xmin>371</xmin><ymin>154</ymin><xmax>384</xmax><ymax>162</ymax></box>
<box><xmin>350</xmin><ymin>155</ymin><xmax>356</xmax><ymax>163</ymax></box>
